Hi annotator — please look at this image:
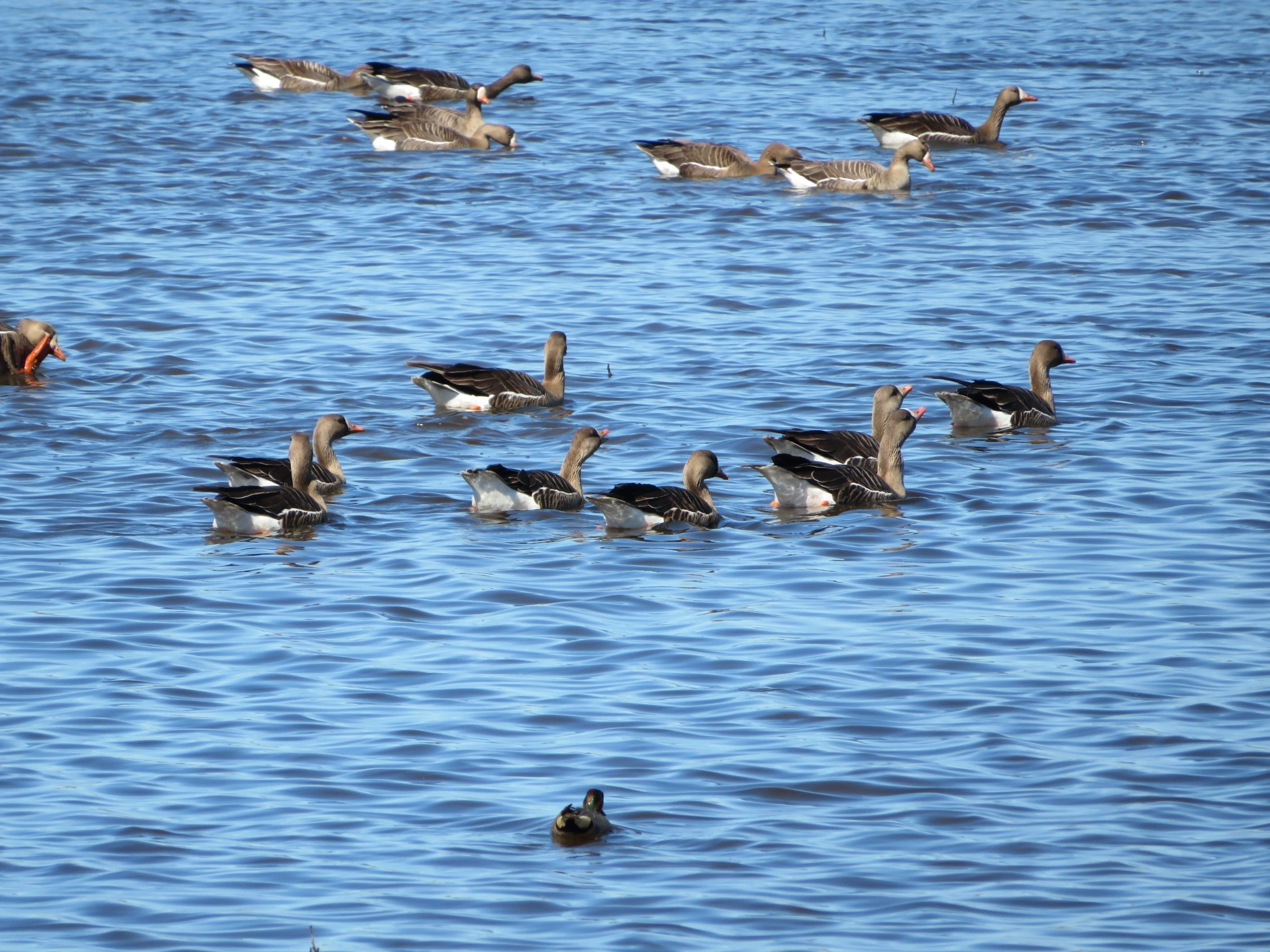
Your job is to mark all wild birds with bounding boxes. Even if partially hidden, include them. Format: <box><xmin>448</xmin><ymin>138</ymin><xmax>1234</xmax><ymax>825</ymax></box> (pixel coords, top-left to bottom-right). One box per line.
<box><xmin>744</xmin><ymin>384</ymin><xmax>926</xmax><ymax>508</ymax></box>
<box><xmin>347</xmin><ymin>58</ymin><xmax>543</xmax><ymax>152</ymax></box>
<box><xmin>767</xmin><ymin>139</ymin><xmax>936</xmax><ymax>190</ymax></box>
<box><xmin>231</xmin><ymin>53</ymin><xmax>373</xmax><ymax>93</ymax></box>
<box><xmin>554</xmin><ymin>789</ymin><xmax>612</xmax><ymax>837</ymax></box>
<box><xmin>933</xmin><ymin>340</ymin><xmax>1075</xmax><ymax>430</ymax></box>
<box><xmin>857</xmin><ymin>85</ymin><xmax>1039</xmax><ymax>147</ymax></box>
<box><xmin>0</xmin><ymin>318</ymin><xmax>66</xmax><ymax>374</ymax></box>
<box><xmin>584</xmin><ymin>446</ymin><xmax>728</xmax><ymax>529</ymax></box>
<box><xmin>407</xmin><ymin>330</ymin><xmax>568</xmax><ymax>413</ymax></box>
<box><xmin>457</xmin><ymin>425</ymin><xmax>610</xmax><ymax>513</ymax></box>
<box><xmin>633</xmin><ymin>139</ymin><xmax>800</xmax><ymax>178</ymax></box>
<box><xmin>192</xmin><ymin>411</ymin><xmax>365</xmax><ymax>534</ymax></box>
